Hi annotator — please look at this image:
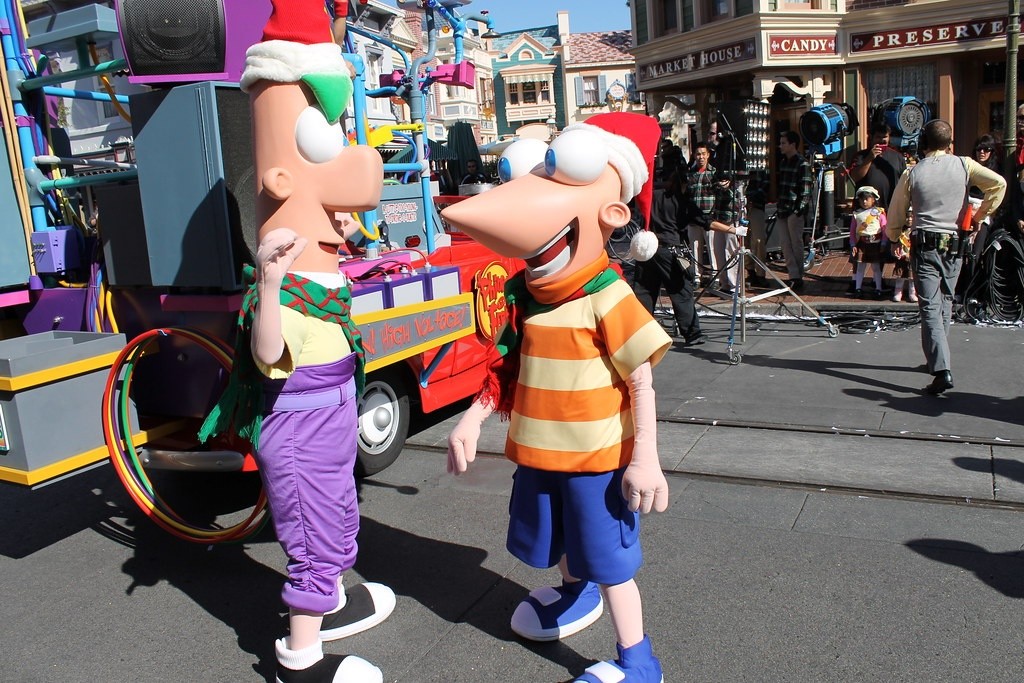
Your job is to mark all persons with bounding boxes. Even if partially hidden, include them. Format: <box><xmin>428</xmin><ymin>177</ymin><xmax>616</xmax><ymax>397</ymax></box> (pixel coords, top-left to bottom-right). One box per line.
<box><xmin>460</xmin><ymin>160</ymin><xmax>491</xmax><ymax>184</ymax></box>
<box><xmin>635</xmin><ymin>141</ymin><xmax>767</xmax><ymax>345</ymax></box>
<box><xmin>887</xmin><ymin>119</ymin><xmax>1007</xmax><ymax>395</ymax></box>
<box><xmin>239</xmin><ymin>0</ymin><xmax>395</xmax><ymax>683</ymax></box>
<box><xmin>952</xmin><ymin>134</ymin><xmax>999</xmax><ymax>305</ymax></box>
<box><xmin>986</xmin><ymin>104</ymin><xmax>1024</xmax><ymax>305</ymax></box>
<box><xmin>777</xmin><ymin>131</ymin><xmax>811</xmax><ymax>294</ymax></box>
<box><xmin>891</xmin><ymin>207</ymin><xmax>918</xmax><ymax>302</ymax></box>
<box><xmin>849</xmin><ymin>186</ymin><xmax>887</xmax><ymax>297</ymax></box>
<box><xmin>844</xmin><ymin>125</ymin><xmax>907</xmax><ymax>296</ymax></box>
<box><xmin>439</xmin><ymin>112</ymin><xmax>674</xmax><ymax>681</ymax></box>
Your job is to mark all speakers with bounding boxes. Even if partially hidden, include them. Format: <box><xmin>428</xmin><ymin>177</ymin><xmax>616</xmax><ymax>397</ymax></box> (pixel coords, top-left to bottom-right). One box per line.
<box><xmin>114</xmin><ymin>0</ymin><xmax>282</xmax><ymax>291</ymax></box>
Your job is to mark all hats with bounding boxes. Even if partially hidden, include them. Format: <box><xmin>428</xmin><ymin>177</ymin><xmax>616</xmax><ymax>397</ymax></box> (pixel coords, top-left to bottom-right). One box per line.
<box><xmin>855</xmin><ymin>185</ymin><xmax>881</xmax><ymax>201</ymax></box>
<box><xmin>554</xmin><ymin>108</ymin><xmax>665</xmax><ymax>261</ymax></box>
<box><xmin>237</xmin><ymin>0</ymin><xmax>352</xmax><ymax>93</ymax></box>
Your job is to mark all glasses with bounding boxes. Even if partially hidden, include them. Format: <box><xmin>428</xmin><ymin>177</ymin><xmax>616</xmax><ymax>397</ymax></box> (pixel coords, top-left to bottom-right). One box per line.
<box><xmin>1016</xmin><ymin>115</ymin><xmax>1024</xmax><ymax>121</ymax></box>
<box><xmin>976</xmin><ymin>147</ymin><xmax>993</xmax><ymax>152</ymax></box>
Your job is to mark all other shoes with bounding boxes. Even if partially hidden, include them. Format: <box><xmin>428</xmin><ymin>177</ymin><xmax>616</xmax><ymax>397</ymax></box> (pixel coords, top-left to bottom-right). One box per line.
<box><xmin>908</xmin><ymin>289</ymin><xmax>919</xmax><ymax>302</ymax></box>
<box><xmin>872</xmin><ymin>289</ymin><xmax>883</xmax><ymax>300</ymax></box>
<box><xmin>781</xmin><ymin>277</ymin><xmax>804</xmax><ymax>290</ymax></box>
<box><xmin>853</xmin><ymin>288</ymin><xmax>864</xmax><ymax>299</ymax></box>
<box><xmin>892</xmin><ymin>288</ymin><xmax>903</xmax><ymax>302</ymax></box>
<box><xmin>744</xmin><ymin>274</ymin><xmax>770</xmax><ymax>288</ymax></box>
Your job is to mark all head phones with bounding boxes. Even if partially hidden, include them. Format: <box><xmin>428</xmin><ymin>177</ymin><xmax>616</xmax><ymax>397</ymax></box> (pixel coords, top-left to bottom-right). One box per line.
<box><xmin>918</xmin><ymin>119</ymin><xmax>952</xmax><ymax>150</ymax></box>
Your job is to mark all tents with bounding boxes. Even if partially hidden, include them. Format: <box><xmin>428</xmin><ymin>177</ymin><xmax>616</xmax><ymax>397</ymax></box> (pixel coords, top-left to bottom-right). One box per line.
<box><xmin>478</xmin><ymin>138</ymin><xmax>515</xmax><ymax>162</ymax></box>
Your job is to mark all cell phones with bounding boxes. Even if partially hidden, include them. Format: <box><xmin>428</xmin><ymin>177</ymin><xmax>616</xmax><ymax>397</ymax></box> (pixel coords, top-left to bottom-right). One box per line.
<box><xmin>876</xmin><ymin>145</ymin><xmax>887</xmax><ymax>151</ymax></box>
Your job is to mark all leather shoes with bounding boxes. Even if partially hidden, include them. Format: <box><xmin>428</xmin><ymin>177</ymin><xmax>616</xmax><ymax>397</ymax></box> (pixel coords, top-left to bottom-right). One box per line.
<box><xmin>925</xmin><ymin>370</ymin><xmax>954</xmax><ymax>394</ymax></box>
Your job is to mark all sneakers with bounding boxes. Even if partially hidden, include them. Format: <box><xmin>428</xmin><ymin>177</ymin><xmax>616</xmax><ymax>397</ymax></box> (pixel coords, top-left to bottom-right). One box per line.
<box><xmin>687</xmin><ymin>335</ymin><xmax>708</xmax><ymax>346</ymax></box>
